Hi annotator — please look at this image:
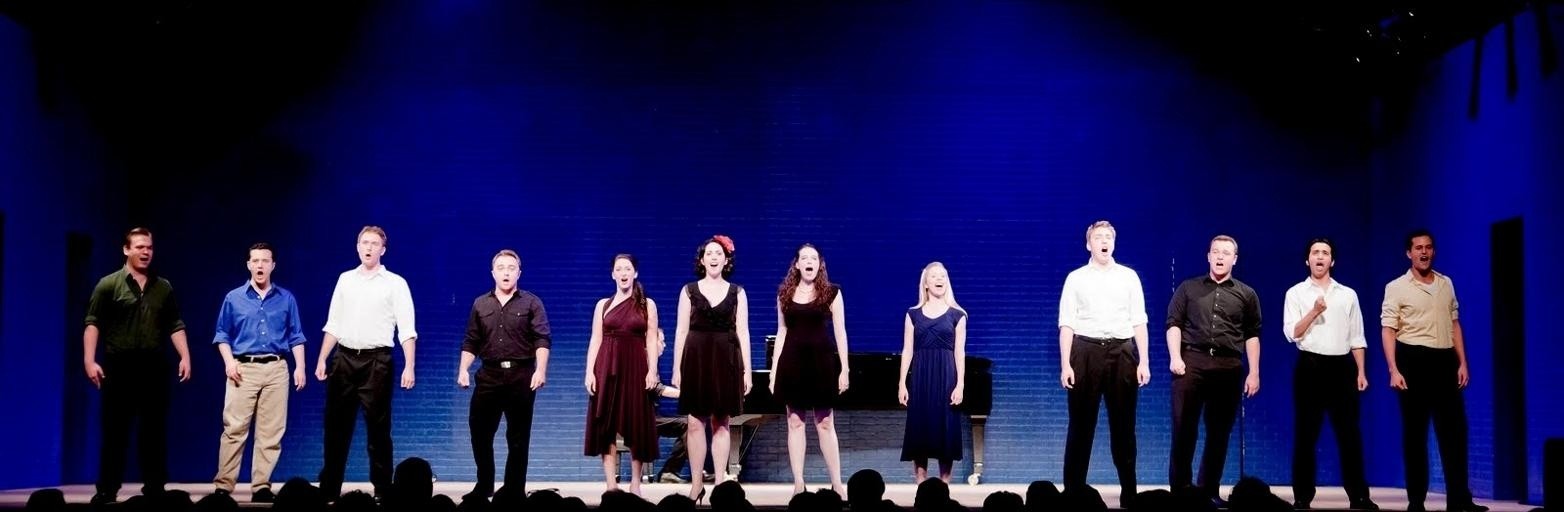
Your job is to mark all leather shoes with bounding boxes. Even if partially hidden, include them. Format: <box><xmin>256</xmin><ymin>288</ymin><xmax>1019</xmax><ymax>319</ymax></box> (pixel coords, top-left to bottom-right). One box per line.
<box><xmin>251</xmin><ymin>489</ymin><xmax>276</xmax><ymax>502</ymax></box>
<box><xmin>658</xmin><ymin>470</ymin><xmax>688</xmax><ymax>483</ymax></box>
<box><xmin>1447</xmin><ymin>501</ymin><xmax>1489</xmax><ymax>512</ymax></box>
<box><xmin>1351</xmin><ymin>497</ymin><xmax>1378</xmax><ymax>511</ymax></box>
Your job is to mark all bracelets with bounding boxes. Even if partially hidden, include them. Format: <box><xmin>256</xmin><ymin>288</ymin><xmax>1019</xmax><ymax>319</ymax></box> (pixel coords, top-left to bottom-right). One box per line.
<box><xmin>743</xmin><ymin>368</ymin><xmax>752</xmax><ymax>373</ymax></box>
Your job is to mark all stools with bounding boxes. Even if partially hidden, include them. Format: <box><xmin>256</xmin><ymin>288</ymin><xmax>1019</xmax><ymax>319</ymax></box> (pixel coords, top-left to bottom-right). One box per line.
<box><xmin>614</xmin><ymin>430</ymin><xmax>654</xmax><ymax>482</ymax></box>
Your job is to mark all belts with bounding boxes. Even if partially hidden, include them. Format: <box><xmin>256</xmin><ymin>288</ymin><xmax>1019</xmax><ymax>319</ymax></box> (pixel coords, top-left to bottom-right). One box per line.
<box><xmin>238</xmin><ymin>355</ymin><xmax>283</xmax><ymax>363</ymax></box>
<box><xmin>339</xmin><ymin>350</ymin><xmax>384</xmax><ymax>355</ymax></box>
<box><xmin>1185</xmin><ymin>346</ymin><xmax>1240</xmax><ymax>357</ymax></box>
<box><xmin>482</xmin><ymin>358</ymin><xmax>534</xmax><ymax>369</ymax></box>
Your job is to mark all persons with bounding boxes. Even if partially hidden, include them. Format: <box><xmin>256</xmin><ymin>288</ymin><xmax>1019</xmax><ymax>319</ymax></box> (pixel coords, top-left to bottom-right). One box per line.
<box><xmin>669</xmin><ymin>233</ymin><xmax>757</xmax><ymax>505</ymax></box>
<box><xmin>1282</xmin><ymin>234</ymin><xmax>1379</xmax><ymax>511</ymax></box>
<box><xmin>317</xmin><ymin>225</ymin><xmax>417</xmax><ymax>499</ymax></box>
<box><xmin>82</xmin><ymin>225</ymin><xmax>193</xmax><ymax>503</ymax></box>
<box><xmin>213</xmin><ymin>241</ymin><xmax>307</xmax><ymax>506</ymax></box>
<box><xmin>24</xmin><ymin>456</ymin><xmax>1297</xmax><ymax>510</ymax></box>
<box><xmin>1377</xmin><ymin>229</ymin><xmax>1489</xmax><ymax>511</ymax></box>
<box><xmin>583</xmin><ymin>253</ymin><xmax>662</xmax><ymax>497</ymax></box>
<box><xmin>614</xmin><ymin>325</ymin><xmax>717</xmax><ymax>484</ymax></box>
<box><xmin>765</xmin><ymin>243</ymin><xmax>850</xmax><ymax>503</ymax></box>
<box><xmin>455</xmin><ymin>248</ymin><xmax>552</xmax><ymax>500</ymax></box>
<box><xmin>895</xmin><ymin>260</ymin><xmax>969</xmax><ymax>486</ymax></box>
<box><xmin>1056</xmin><ymin>219</ymin><xmax>1153</xmax><ymax>507</ymax></box>
<box><xmin>1162</xmin><ymin>235</ymin><xmax>1263</xmax><ymax>507</ymax></box>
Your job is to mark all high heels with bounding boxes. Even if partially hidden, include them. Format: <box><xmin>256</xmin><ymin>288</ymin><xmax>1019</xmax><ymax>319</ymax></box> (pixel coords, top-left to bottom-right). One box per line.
<box><xmin>689</xmin><ymin>486</ymin><xmax>706</xmax><ymax>506</ymax></box>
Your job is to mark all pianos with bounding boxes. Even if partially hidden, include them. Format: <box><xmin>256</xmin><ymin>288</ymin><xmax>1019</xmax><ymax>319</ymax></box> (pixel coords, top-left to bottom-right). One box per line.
<box><xmin>727</xmin><ymin>334</ymin><xmax>992</xmax><ymax>483</ymax></box>
